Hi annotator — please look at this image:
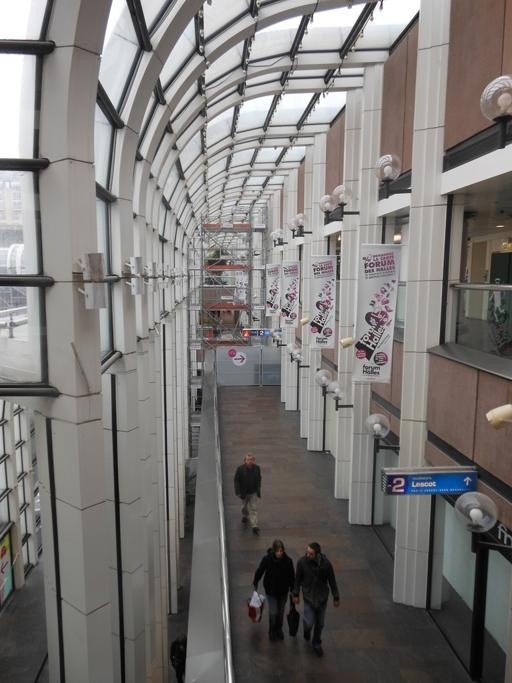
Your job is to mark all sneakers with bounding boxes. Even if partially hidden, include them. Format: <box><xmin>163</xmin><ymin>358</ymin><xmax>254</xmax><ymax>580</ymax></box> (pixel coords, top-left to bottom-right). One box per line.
<box><xmin>239</xmin><ymin>516</ymin><xmax>263</xmax><ymax>537</ymax></box>
<box><xmin>315</xmin><ymin>647</ymin><xmax>324</xmax><ymax>659</ymax></box>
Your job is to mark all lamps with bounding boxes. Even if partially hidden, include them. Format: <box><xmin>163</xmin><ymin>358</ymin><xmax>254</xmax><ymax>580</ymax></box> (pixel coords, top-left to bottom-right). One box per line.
<box><xmin>373</xmin><ymin>152</ymin><xmax>411</xmax><ymax>200</ymax></box>
<box><xmin>453</xmin><ymin>492</ymin><xmax>511</xmax><ymax>558</ymax></box>
<box><xmin>270</xmin><ymin>184</ymin><xmax>359</xmax><ymax>247</ymax></box>
<box><xmin>479</xmin><ymin>73</ymin><xmax>512</xmax><ymax>149</ymax></box>
<box><xmin>483</xmin><ymin>402</ymin><xmax>511</xmax><ymax>428</ymax></box>
<box><xmin>269</xmin><ymin>316</ymin><xmax>354</xmax><ymax>411</ymax></box>
<box><xmin>364</xmin><ymin>413</ymin><xmax>400</xmax><ymax>453</ymax></box>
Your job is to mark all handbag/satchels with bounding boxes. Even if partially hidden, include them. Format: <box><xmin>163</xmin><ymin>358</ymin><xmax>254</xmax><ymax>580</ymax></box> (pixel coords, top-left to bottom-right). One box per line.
<box><xmin>245</xmin><ymin>589</ymin><xmax>266</xmax><ymax>623</ymax></box>
<box><xmin>286</xmin><ymin>594</ymin><xmax>300</xmax><ymax>636</ymax></box>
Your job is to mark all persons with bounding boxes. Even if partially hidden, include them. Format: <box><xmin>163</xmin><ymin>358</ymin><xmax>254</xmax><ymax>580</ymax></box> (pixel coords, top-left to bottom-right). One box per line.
<box><xmin>233</xmin><ymin>452</ymin><xmax>264</xmax><ymax>532</ymax></box>
<box><xmin>291</xmin><ymin>541</ymin><xmax>340</xmax><ymax>656</ymax></box>
<box><xmin>253</xmin><ymin>539</ymin><xmax>295</xmax><ymax>643</ymax></box>
<box><xmin>171</xmin><ymin>626</ymin><xmax>188</xmax><ymax>682</ymax></box>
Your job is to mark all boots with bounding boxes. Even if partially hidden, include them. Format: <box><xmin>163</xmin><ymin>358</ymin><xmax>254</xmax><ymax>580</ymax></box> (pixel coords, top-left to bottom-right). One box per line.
<box><xmin>268</xmin><ymin>613</ymin><xmax>285</xmax><ymax>642</ymax></box>
